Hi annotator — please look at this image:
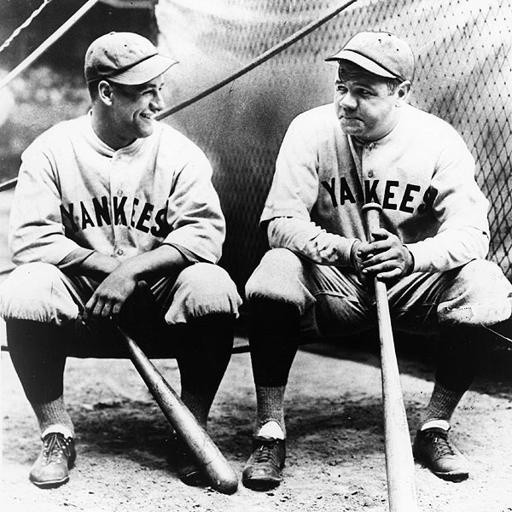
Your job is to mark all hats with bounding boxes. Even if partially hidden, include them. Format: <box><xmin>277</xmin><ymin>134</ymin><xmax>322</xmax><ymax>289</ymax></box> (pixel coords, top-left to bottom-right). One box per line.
<box><xmin>81</xmin><ymin>29</ymin><xmax>182</xmax><ymax>86</ymax></box>
<box><xmin>324</xmin><ymin>29</ymin><xmax>417</xmax><ymax>92</ymax></box>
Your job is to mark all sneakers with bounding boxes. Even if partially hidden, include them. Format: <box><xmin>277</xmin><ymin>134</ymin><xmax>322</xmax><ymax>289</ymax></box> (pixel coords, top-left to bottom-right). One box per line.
<box><xmin>412</xmin><ymin>415</ymin><xmax>470</xmax><ymax>477</ymax></box>
<box><xmin>28</xmin><ymin>421</ymin><xmax>79</xmax><ymax>487</ymax></box>
<box><xmin>172</xmin><ymin>424</ymin><xmax>212</xmax><ymax>488</ymax></box>
<box><xmin>240</xmin><ymin>429</ymin><xmax>287</xmax><ymax>487</ymax></box>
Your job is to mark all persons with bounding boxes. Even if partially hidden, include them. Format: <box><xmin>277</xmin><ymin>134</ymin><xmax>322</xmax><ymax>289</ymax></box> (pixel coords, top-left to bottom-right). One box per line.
<box><xmin>241</xmin><ymin>27</ymin><xmax>510</xmax><ymax>492</ymax></box>
<box><xmin>0</xmin><ymin>28</ymin><xmax>244</xmax><ymax>490</ymax></box>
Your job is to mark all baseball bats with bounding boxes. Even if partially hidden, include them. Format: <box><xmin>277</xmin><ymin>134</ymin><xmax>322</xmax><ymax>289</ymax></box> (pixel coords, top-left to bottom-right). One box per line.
<box><xmin>80</xmin><ymin>274</ymin><xmax>238</xmax><ymax>494</ymax></box>
<box><xmin>362</xmin><ymin>203</ymin><xmax>419</xmax><ymax>512</ymax></box>
